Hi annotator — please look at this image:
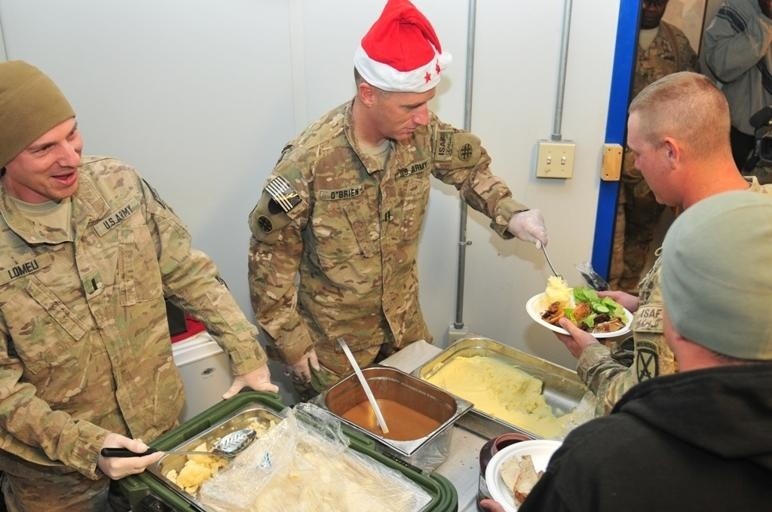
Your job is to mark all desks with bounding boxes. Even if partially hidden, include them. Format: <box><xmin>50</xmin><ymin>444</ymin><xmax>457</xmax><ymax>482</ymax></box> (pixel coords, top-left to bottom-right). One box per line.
<box><xmin>379</xmin><ymin>340</ymin><xmax>555</xmax><ymax>511</ymax></box>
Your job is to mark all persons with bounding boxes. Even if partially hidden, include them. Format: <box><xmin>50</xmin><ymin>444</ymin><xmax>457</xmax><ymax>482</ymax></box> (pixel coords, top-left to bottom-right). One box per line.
<box><xmin>478</xmin><ymin>188</ymin><xmax>771</xmax><ymax>512</ymax></box>
<box><xmin>617</xmin><ymin>1</ymin><xmax>696</xmax><ymax>290</ymax></box>
<box><xmin>246</xmin><ymin>0</ymin><xmax>550</xmax><ymax>402</ymax></box>
<box><xmin>697</xmin><ymin>1</ymin><xmax>771</xmax><ymax>172</ymax></box>
<box><xmin>551</xmin><ymin>70</ymin><xmax>771</xmax><ymax>420</ymax></box>
<box><xmin>1</xmin><ymin>57</ymin><xmax>279</xmax><ymax>512</ymax></box>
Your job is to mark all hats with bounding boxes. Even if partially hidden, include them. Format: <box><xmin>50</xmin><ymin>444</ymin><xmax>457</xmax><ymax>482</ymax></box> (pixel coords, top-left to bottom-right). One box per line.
<box><xmin>0</xmin><ymin>60</ymin><xmax>78</xmax><ymax>169</ymax></box>
<box><xmin>662</xmin><ymin>190</ymin><xmax>772</xmax><ymax>361</ymax></box>
<box><xmin>353</xmin><ymin>0</ymin><xmax>453</xmax><ymax>94</ymax></box>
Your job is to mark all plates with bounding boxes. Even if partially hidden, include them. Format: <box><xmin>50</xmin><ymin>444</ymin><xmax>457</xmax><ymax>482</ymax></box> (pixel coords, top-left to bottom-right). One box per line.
<box><xmin>525</xmin><ymin>290</ymin><xmax>636</xmax><ymax>340</ymax></box>
<box><xmin>484</xmin><ymin>440</ymin><xmax>581</xmax><ymax>512</ymax></box>
